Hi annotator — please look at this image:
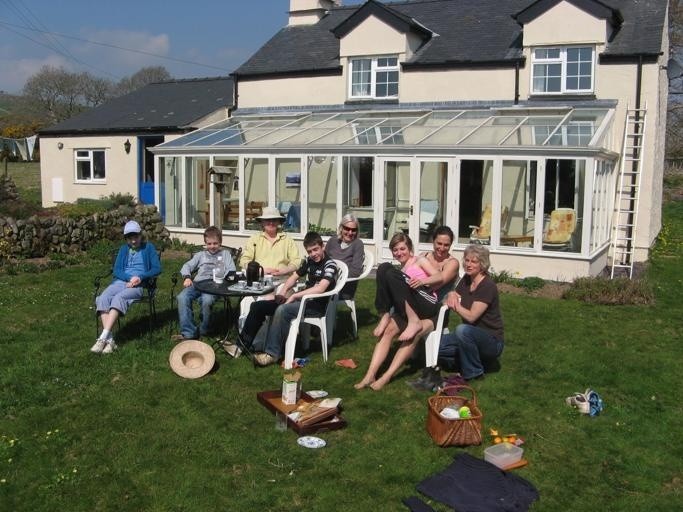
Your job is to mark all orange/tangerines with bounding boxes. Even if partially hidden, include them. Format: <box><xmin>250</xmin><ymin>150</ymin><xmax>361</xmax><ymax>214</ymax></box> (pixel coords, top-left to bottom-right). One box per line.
<box><xmin>494</xmin><ymin>437</ymin><xmax>515</xmax><ymax>444</ymax></box>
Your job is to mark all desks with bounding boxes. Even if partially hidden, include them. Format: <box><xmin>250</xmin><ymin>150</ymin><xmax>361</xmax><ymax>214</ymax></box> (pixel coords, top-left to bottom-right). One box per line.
<box><xmin>497</xmin><ymin>233</ymin><xmax>534</xmax><ymax>248</ymax></box>
<box><xmin>187</xmin><ymin>275</ymin><xmax>279</xmax><ymax>367</ymax></box>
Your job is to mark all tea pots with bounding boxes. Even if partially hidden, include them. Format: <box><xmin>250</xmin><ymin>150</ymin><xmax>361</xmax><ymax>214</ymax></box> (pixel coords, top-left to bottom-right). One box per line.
<box><xmin>246</xmin><ymin>262</ymin><xmax>264</xmax><ymax>285</ymax></box>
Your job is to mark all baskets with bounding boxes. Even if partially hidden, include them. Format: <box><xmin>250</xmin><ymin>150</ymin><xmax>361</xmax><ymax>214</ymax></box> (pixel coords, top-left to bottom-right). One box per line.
<box><xmin>426</xmin><ymin>384</ymin><xmax>484</xmax><ymax>448</ymax></box>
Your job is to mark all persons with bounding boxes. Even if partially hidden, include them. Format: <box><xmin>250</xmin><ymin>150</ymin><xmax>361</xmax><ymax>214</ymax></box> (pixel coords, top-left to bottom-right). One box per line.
<box><xmin>353</xmin><ymin>224</ymin><xmax>459</xmax><ymax>390</ymax></box>
<box><xmin>321</xmin><ymin>213</ymin><xmax>365</xmax><ymax>280</ymax></box>
<box><xmin>237</xmin><ymin>205</ymin><xmax>301</xmax><ymax>352</ymax></box>
<box><xmin>437</xmin><ymin>244</ymin><xmax>505</xmax><ymax>383</ymax></box>
<box><xmin>214</xmin><ymin>232</ymin><xmax>337</xmax><ymax>368</ymax></box>
<box><xmin>371</xmin><ymin>233</ymin><xmax>443</xmax><ymax>344</ymax></box>
<box><xmin>168</xmin><ymin>226</ymin><xmax>237</xmax><ymax>340</ymax></box>
<box><xmin>88</xmin><ymin>221</ymin><xmax>160</xmax><ymax>357</ymax></box>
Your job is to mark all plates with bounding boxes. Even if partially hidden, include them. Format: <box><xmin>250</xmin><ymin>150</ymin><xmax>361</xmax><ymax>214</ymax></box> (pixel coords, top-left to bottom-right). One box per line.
<box><xmin>304</xmin><ymin>390</ymin><xmax>328</xmax><ymax>398</ymax></box>
<box><xmin>296</xmin><ymin>436</ymin><xmax>326</xmax><ymax>450</ymax></box>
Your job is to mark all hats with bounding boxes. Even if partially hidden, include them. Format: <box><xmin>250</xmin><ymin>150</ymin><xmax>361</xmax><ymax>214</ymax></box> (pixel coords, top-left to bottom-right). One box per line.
<box><xmin>255</xmin><ymin>206</ymin><xmax>286</xmax><ymax>222</ymax></box>
<box><xmin>123</xmin><ymin>221</ymin><xmax>141</xmax><ymax>238</ymax></box>
<box><xmin>169</xmin><ymin>340</ymin><xmax>215</xmax><ymax>380</ymax></box>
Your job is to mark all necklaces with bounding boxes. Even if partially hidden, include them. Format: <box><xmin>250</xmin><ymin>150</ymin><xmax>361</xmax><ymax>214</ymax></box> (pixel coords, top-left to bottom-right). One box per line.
<box><xmin>469</xmin><ymin>276</ymin><xmax>482</xmax><ymax>289</ymax></box>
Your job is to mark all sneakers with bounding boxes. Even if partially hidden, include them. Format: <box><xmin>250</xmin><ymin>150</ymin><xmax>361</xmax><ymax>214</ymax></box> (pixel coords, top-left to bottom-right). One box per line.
<box><xmin>214</xmin><ymin>337</ymin><xmax>244</xmax><ymax>360</ymax></box>
<box><xmin>463</xmin><ymin>372</ymin><xmax>486</xmax><ymax>384</ymax></box>
<box><xmin>171</xmin><ymin>334</ymin><xmax>196</xmax><ymax>345</ymax></box>
<box><xmin>441</xmin><ymin>376</ymin><xmax>467</xmax><ymax>393</ymax></box>
<box><xmin>407</xmin><ymin>367</ymin><xmax>441</xmax><ymax>391</ymax></box>
<box><xmin>90</xmin><ymin>337</ymin><xmax>118</xmax><ymax>354</ymax></box>
<box><xmin>253</xmin><ymin>352</ymin><xmax>280</xmax><ymax>367</ymax></box>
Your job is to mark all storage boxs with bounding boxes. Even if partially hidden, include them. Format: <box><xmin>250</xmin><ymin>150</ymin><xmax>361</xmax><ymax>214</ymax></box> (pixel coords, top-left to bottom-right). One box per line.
<box><xmin>483</xmin><ymin>442</ymin><xmax>523</xmax><ymax>467</ymax></box>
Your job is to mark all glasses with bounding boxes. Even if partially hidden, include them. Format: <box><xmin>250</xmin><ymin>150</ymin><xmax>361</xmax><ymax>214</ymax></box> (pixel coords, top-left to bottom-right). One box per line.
<box><xmin>341</xmin><ymin>224</ymin><xmax>358</xmax><ymax>231</ymax></box>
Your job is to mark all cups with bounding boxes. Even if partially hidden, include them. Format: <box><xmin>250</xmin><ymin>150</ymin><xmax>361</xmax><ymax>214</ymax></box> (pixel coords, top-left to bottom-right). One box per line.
<box><xmin>235</xmin><ymin>272</ymin><xmax>273</xmax><ymax>290</ymax></box>
<box><xmin>274</xmin><ymin>411</ymin><xmax>288</xmax><ymax>432</ymax></box>
<box><xmin>212</xmin><ymin>268</ymin><xmax>220</xmax><ymax>282</ymax></box>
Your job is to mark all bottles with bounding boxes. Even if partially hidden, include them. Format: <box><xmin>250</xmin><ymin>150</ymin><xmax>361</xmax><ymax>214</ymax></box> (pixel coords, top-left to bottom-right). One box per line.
<box><xmin>214</xmin><ymin>256</ymin><xmax>224</xmax><ymax>284</ymax></box>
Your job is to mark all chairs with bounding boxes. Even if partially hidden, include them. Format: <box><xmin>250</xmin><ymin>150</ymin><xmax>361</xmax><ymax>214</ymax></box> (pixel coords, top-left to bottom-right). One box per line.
<box><xmin>275</xmin><ymin>258</ymin><xmax>348</xmax><ymax>368</ymax></box>
<box><xmin>402</xmin><ymin>197</ymin><xmax>439</xmax><ymax>242</ymax></box>
<box><xmin>340</xmin><ymin>247</ymin><xmax>372</xmax><ymax>336</ymax></box>
<box><xmin>536</xmin><ymin>206</ymin><xmax>578</xmax><ymax>252</ymax></box>
<box><xmin>92</xmin><ymin>246</ymin><xmax>157</xmax><ymax>348</ymax></box>
<box><xmin>205</xmin><ymin>198</ymin><xmax>291</xmax><ymax>232</ymax></box>
<box><xmin>169</xmin><ymin>242</ymin><xmax>242</xmax><ymax>336</ymax></box>
<box><xmin>418</xmin><ymin>262</ymin><xmax>472</xmax><ymax>367</ymax></box>
<box><xmin>468</xmin><ymin>205</ymin><xmax>508</xmax><ymax>247</ymax></box>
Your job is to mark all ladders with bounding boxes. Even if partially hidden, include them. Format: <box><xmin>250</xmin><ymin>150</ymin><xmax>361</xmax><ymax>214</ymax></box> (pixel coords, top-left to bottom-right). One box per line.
<box><xmin>610</xmin><ymin>100</ymin><xmax>648</xmax><ymax>279</ymax></box>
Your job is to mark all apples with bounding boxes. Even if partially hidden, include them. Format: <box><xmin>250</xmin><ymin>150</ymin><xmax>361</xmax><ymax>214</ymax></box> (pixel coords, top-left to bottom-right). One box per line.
<box><xmin>460</xmin><ymin>411</ymin><xmax>469</xmax><ymax>417</ymax></box>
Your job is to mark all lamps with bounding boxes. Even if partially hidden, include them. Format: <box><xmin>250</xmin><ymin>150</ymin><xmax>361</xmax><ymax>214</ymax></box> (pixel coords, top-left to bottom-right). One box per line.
<box><xmin>122</xmin><ymin>139</ymin><xmax>131</xmax><ymax>154</ymax></box>
